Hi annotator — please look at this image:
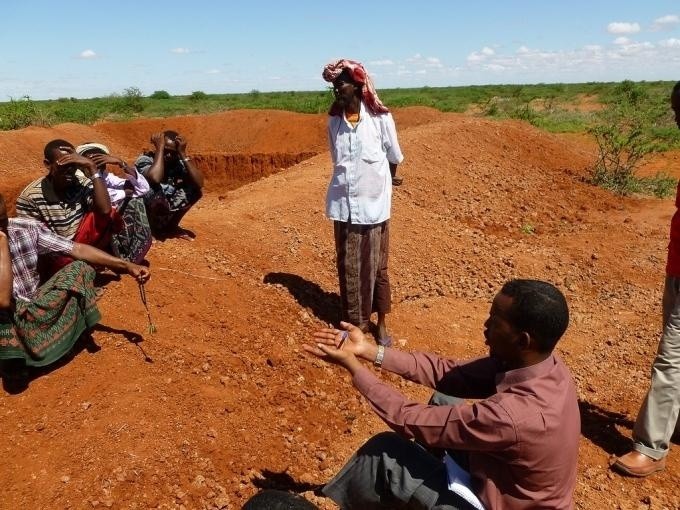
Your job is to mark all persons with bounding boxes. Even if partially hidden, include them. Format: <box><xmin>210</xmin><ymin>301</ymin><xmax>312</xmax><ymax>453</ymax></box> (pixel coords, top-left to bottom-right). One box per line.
<box><xmin>615</xmin><ymin>79</ymin><xmax>680</xmax><ymax>478</ymax></box>
<box><xmin>300</xmin><ymin>279</ymin><xmax>582</xmax><ymax>510</ymax></box>
<box><xmin>16</xmin><ymin>139</ymin><xmax>124</xmax><ymax>276</ymax></box>
<box><xmin>1</xmin><ymin>192</ymin><xmax>151</xmax><ymax>366</ymax></box>
<box><xmin>75</xmin><ymin>143</ymin><xmax>153</xmax><ymax>265</ymax></box>
<box><xmin>321</xmin><ymin>58</ymin><xmax>404</xmax><ymax>348</ymax></box>
<box><xmin>134</xmin><ymin>131</ymin><xmax>205</xmax><ymax>241</ymax></box>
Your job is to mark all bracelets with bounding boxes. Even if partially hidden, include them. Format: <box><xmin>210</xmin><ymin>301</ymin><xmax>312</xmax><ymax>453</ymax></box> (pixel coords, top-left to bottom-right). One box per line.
<box><xmin>91</xmin><ymin>173</ymin><xmax>102</xmax><ymax>180</ymax></box>
<box><xmin>373</xmin><ymin>344</ymin><xmax>385</xmax><ymax>366</ymax></box>
<box><xmin>183</xmin><ymin>156</ymin><xmax>192</xmax><ymax>162</ymax></box>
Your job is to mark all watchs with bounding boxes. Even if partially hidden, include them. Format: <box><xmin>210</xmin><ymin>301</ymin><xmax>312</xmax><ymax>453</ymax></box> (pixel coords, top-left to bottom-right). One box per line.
<box><xmin>119</xmin><ymin>160</ymin><xmax>128</xmax><ymax>170</ymax></box>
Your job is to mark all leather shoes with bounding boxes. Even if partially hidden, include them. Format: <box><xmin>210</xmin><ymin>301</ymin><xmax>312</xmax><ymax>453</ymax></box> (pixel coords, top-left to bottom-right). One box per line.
<box><xmin>615</xmin><ymin>448</ymin><xmax>666</xmax><ymax>477</ymax></box>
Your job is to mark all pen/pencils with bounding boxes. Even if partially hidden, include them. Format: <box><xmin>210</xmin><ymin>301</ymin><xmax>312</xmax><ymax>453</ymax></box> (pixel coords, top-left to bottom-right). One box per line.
<box><xmin>342</xmin><ymin>331</ymin><xmax>348</xmax><ymax>340</ymax></box>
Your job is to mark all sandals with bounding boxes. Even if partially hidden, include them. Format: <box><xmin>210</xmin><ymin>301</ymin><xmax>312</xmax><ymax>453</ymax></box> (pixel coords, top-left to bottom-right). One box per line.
<box><xmin>377</xmin><ymin>335</ymin><xmax>392</xmax><ymax>348</ymax></box>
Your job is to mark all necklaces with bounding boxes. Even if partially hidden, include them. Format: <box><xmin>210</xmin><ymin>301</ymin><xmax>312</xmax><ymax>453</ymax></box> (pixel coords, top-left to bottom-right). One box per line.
<box><xmin>136</xmin><ymin>275</ymin><xmax>157</xmax><ymax>333</ymax></box>
